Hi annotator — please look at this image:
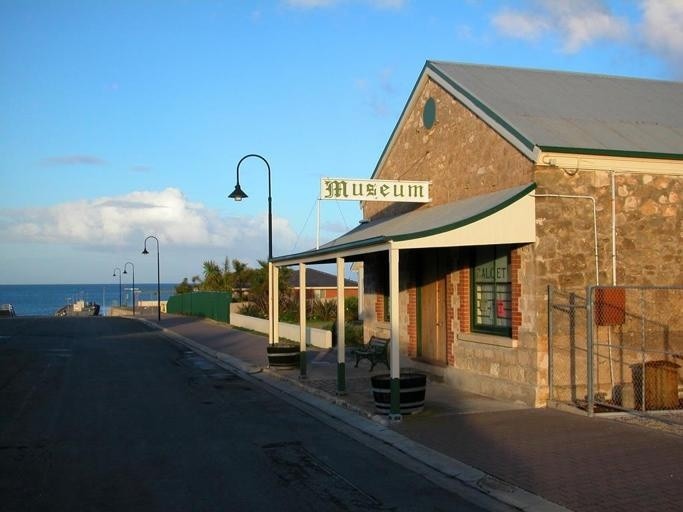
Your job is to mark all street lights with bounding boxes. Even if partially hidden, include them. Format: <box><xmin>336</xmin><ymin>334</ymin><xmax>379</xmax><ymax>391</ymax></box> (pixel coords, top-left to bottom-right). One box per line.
<box><xmin>142</xmin><ymin>235</ymin><xmax>162</xmax><ymax>324</ymax></box>
<box><xmin>227</xmin><ymin>155</ymin><xmax>277</xmax><ymax>350</ymax></box>
<box><xmin>112</xmin><ymin>261</ymin><xmax>137</xmax><ymax>316</ymax></box>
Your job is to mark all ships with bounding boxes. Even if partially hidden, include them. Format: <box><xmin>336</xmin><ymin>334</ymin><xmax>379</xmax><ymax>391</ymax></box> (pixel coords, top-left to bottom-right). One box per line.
<box><xmin>54</xmin><ymin>288</ymin><xmax>100</xmax><ymax>317</ymax></box>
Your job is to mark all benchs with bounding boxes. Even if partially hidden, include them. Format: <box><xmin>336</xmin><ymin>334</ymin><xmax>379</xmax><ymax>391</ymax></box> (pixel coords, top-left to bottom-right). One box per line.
<box><xmin>351</xmin><ymin>336</ymin><xmax>391</xmax><ymax>372</ymax></box>
